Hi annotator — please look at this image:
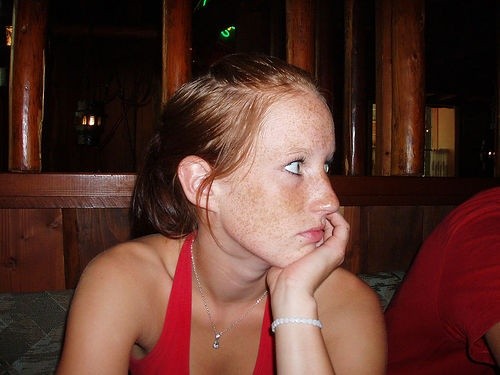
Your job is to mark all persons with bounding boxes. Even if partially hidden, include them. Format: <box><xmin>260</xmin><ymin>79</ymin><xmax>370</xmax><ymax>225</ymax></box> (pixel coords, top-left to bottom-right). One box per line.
<box><xmin>56</xmin><ymin>51</ymin><xmax>387</xmax><ymax>375</ymax></box>
<box><xmin>383</xmin><ymin>185</ymin><xmax>500</xmax><ymax>374</ymax></box>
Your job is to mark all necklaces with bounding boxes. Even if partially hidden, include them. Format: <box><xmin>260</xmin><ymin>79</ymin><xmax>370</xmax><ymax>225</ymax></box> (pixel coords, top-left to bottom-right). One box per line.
<box><xmin>189</xmin><ymin>236</ymin><xmax>270</xmax><ymax>349</ymax></box>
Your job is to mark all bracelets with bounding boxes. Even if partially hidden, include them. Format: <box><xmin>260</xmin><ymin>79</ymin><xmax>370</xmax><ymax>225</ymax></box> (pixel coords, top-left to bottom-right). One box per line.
<box><xmin>272</xmin><ymin>316</ymin><xmax>322</xmax><ymax>334</ymax></box>
